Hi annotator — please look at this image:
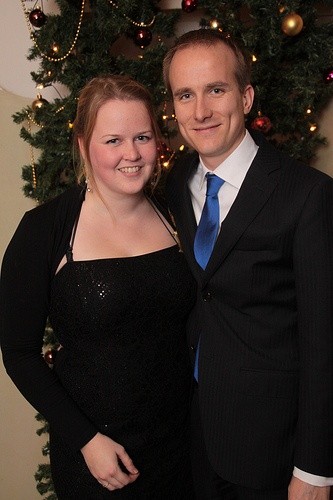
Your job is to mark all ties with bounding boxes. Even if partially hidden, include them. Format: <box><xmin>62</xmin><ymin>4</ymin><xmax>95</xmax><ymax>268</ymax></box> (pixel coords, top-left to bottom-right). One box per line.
<box><xmin>194</xmin><ymin>172</ymin><xmax>225</xmax><ymax>383</ymax></box>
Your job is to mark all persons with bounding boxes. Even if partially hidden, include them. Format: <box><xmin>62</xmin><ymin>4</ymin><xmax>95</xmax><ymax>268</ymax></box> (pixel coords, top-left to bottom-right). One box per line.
<box><xmin>0</xmin><ymin>75</ymin><xmax>199</xmax><ymax>499</ymax></box>
<box><xmin>147</xmin><ymin>28</ymin><xmax>333</xmax><ymax>499</ymax></box>
